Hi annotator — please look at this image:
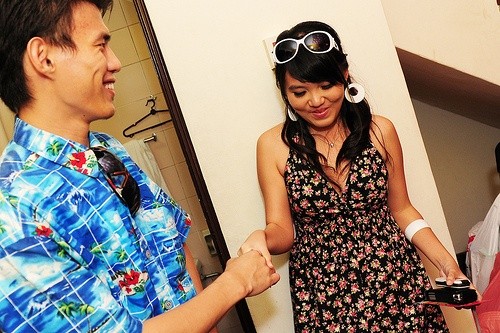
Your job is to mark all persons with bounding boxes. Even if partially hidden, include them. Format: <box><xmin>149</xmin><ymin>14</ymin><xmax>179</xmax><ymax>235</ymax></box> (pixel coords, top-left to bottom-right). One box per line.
<box><xmin>237</xmin><ymin>21</ymin><xmax>482</xmax><ymax>333</ymax></box>
<box><xmin>0</xmin><ymin>0</ymin><xmax>279</xmax><ymax>333</ymax></box>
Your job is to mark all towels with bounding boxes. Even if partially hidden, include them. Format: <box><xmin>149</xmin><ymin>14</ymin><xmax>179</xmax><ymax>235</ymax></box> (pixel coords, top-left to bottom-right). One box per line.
<box><xmin>123</xmin><ymin>138</ymin><xmax>177</xmax><ymax>201</ymax></box>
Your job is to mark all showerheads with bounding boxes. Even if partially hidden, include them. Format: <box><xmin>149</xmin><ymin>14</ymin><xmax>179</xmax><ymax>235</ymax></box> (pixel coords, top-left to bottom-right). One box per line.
<box><xmin>148</xmin><ymin>95</ymin><xmax>159</xmax><ymax>102</ymax></box>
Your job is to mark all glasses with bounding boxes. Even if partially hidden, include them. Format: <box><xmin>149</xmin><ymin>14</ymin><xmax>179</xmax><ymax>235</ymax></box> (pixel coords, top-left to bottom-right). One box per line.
<box><xmin>273</xmin><ymin>31</ymin><xmax>339</xmax><ymax>66</ymax></box>
<box><xmin>93</xmin><ymin>146</ymin><xmax>140</xmax><ymax>217</ymax></box>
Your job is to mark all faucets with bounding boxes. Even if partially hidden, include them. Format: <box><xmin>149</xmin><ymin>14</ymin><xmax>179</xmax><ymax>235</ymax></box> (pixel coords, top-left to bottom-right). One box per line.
<box><xmin>195</xmin><ymin>258</ymin><xmax>222</xmax><ymax>286</ymax></box>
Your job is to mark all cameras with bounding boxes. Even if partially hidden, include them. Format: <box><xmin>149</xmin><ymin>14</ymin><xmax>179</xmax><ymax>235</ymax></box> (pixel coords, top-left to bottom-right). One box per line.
<box><xmin>423</xmin><ymin>287</ymin><xmax>477</xmax><ymax>304</ymax></box>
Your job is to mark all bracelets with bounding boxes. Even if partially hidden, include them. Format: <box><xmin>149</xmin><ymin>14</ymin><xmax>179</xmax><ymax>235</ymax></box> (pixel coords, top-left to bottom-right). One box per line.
<box><xmin>405</xmin><ymin>219</ymin><xmax>431</xmax><ymax>243</ymax></box>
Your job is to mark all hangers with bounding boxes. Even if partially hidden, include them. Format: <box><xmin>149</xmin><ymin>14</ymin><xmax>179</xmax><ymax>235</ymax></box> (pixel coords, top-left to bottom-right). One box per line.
<box><xmin>122</xmin><ymin>98</ymin><xmax>172</xmax><ymax>138</ymax></box>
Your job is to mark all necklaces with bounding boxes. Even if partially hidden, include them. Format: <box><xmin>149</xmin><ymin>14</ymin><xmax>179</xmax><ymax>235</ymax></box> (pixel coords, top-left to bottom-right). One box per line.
<box><xmin>317</xmin><ymin>136</ymin><xmax>338</xmax><ymax>147</ymax></box>
